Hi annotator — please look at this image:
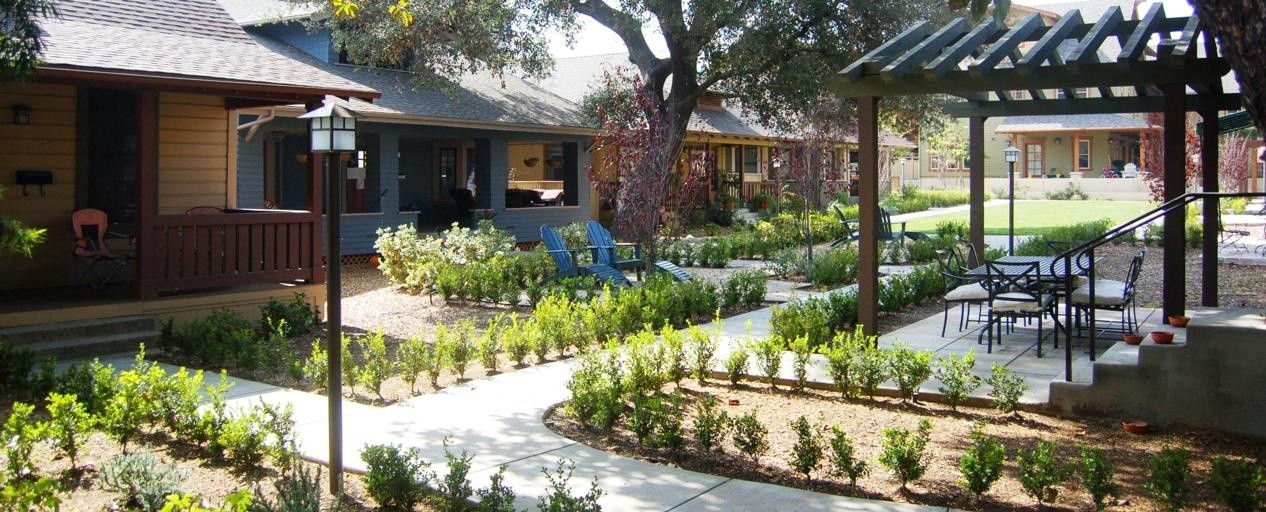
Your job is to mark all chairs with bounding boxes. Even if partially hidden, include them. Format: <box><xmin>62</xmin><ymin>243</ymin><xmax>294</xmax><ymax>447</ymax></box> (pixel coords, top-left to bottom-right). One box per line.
<box><xmin>532</xmin><ymin>214</ymin><xmax>691</xmax><ymax>291</ymax></box>
<box><xmin>829</xmin><ymin>204</ymin><xmax>932</xmax><ymax>261</ymax></box>
<box><xmin>69</xmin><ymin>209</ymin><xmax>135</xmax><ymax>291</ymax></box>
<box><xmin>926</xmin><ymin>240</ymin><xmax>1146</xmax><ymax>355</ymax></box>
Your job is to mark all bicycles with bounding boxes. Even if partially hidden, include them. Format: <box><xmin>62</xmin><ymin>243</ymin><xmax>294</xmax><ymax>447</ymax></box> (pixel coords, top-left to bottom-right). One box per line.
<box><xmin>1100</xmin><ymin>167</ymin><xmax>1119</xmax><ymax>178</ymax></box>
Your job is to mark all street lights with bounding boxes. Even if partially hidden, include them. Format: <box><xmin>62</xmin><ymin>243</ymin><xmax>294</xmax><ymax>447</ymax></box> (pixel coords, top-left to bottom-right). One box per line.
<box><xmin>1004</xmin><ymin>147</ymin><xmax>1022</xmax><ymax>255</ymax></box>
<box><xmin>298</xmin><ymin>103</ymin><xmax>369</xmax><ymax>498</ymax></box>
<box><xmin>772</xmin><ymin>158</ymin><xmax>782</xmax><ymax>215</ymax></box>
